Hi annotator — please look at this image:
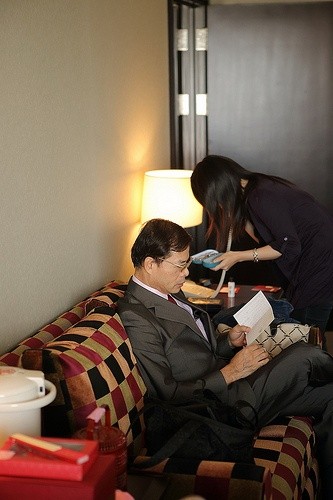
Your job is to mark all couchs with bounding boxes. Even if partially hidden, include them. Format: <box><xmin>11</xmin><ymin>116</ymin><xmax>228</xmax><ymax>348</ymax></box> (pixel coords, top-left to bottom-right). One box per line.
<box><xmin>0</xmin><ymin>279</ymin><xmax>325</xmax><ymax>500</ymax></box>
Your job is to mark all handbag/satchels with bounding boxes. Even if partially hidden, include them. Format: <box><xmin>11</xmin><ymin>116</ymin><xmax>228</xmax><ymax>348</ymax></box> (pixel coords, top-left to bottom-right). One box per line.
<box><xmin>144</xmin><ymin>395</ymin><xmax>258</xmax><ymax>463</ymax></box>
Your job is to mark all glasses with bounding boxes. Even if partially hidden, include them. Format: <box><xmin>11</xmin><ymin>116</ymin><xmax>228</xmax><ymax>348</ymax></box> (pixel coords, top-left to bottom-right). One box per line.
<box><xmin>143</xmin><ymin>255</ymin><xmax>192</xmax><ymax>272</ymax></box>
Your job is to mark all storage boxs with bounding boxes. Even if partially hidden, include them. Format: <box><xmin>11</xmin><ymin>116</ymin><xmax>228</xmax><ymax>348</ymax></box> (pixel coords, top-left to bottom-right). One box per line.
<box><xmin>0</xmin><ymin>432</ymin><xmax>116</xmax><ymax>500</ymax></box>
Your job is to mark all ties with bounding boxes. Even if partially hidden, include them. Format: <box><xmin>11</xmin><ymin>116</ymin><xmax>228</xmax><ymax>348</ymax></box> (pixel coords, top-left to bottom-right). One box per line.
<box><xmin>168</xmin><ymin>294</ymin><xmax>176</xmax><ymax>305</ymax></box>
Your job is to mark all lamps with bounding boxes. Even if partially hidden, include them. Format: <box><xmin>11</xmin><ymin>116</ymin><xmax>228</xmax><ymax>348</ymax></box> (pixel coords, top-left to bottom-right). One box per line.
<box><xmin>140</xmin><ymin>169</ymin><xmax>203</xmax><ymax>229</ymax></box>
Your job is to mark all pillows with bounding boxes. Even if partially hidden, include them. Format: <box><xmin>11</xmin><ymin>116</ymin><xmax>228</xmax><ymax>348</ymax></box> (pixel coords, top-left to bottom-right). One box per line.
<box><xmin>214</xmin><ymin>322</ymin><xmax>311</xmax><ymax>362</ymax></box>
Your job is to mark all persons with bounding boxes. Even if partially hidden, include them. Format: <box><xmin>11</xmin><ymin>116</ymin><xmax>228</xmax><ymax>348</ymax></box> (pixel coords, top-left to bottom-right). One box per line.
<box><xmin>187</xmin><ymin>155</ymin><xmax>332</xmax><ymax>334</ymax></box>
<box><xmin>116</xmin><ymin>217</ymin><xmax>333</xmax><ymax>499</ymax></box>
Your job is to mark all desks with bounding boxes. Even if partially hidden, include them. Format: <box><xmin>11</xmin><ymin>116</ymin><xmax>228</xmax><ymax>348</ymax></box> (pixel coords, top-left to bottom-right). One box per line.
<box><xmin>204</xmin><ymin>284</ymin><xmax>284</xmax><ymax>320</ymax></box>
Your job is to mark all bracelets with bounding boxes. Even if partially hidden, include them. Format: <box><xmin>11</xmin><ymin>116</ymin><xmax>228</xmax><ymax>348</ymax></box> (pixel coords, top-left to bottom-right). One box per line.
<box><xmin>253</xmin><ymin>248</ymin><xmax>259</xmax><ymax>262</ymax></box>
